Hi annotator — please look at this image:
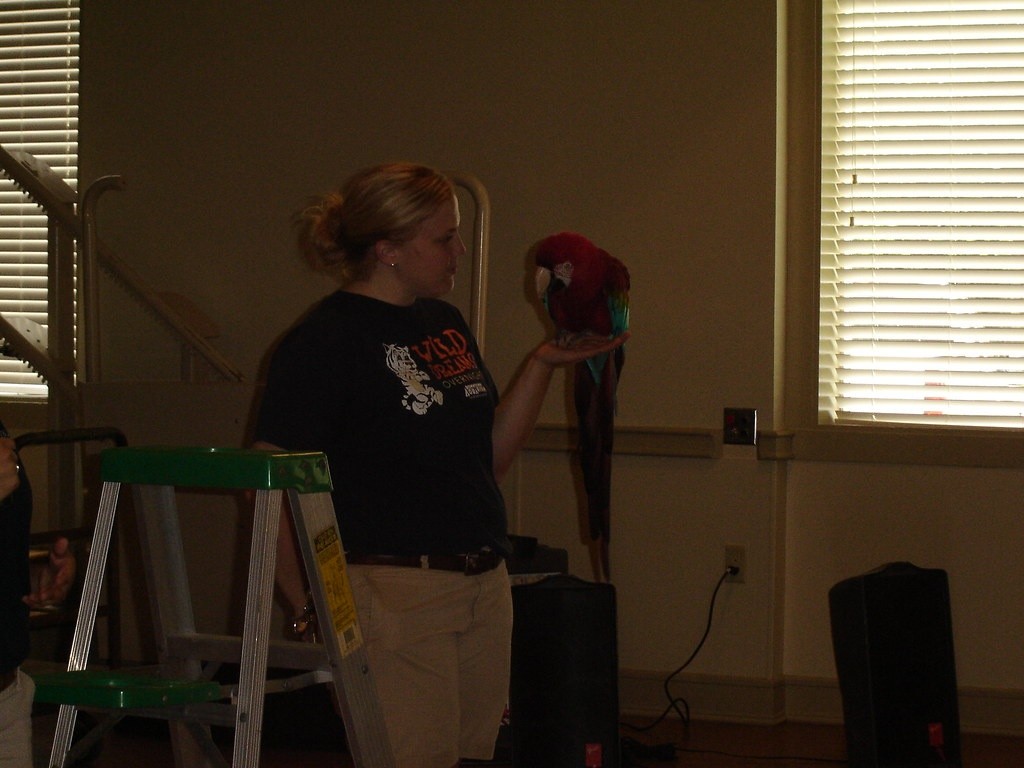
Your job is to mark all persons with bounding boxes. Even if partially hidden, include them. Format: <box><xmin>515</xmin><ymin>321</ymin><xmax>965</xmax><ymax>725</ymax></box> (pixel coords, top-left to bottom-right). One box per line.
<box><xmin>0</xmin><ymin>418</ymin><xmax>37</xmax><ymax>768</ymax></box>
<box><xmin>239</xmin><ymin>160</ymin><xmax>630</xmax><ymax>768</ymax></box>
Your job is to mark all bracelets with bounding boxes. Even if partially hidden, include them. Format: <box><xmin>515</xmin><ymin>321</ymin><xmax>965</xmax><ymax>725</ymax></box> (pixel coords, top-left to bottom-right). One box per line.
<box><xmin>287</xmin><ymin>603</ymin><xmax>319</xmax><ymax>644</ymax></box>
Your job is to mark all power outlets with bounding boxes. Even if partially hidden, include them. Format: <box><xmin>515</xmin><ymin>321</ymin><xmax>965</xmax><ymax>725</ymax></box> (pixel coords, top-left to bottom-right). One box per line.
<box><xmin>726</xmin><ymin>545</ymin><xmax>750</xmax><ymax>585</ymax></box>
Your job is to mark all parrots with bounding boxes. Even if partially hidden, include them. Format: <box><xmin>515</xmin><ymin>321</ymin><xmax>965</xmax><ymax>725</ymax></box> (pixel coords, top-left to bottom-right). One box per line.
<box><xmin>520</xmin><ymin>233</ymin><xmax>631</xmax><ymax>585</ymax></box>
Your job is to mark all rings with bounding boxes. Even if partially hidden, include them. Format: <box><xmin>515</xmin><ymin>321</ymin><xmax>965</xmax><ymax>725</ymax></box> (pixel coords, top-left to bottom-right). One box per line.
<box><xmin>15</xmin><ymin>464</ymin><xmax>21</xmax><ymax>473</ymax></box>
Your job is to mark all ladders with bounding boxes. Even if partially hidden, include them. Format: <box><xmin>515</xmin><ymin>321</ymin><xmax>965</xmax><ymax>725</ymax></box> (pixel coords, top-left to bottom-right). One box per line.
<box><xmin>45</xmin><ymin>440</ymin><xmax>391</xmax><ymax>767</ymax></box>
